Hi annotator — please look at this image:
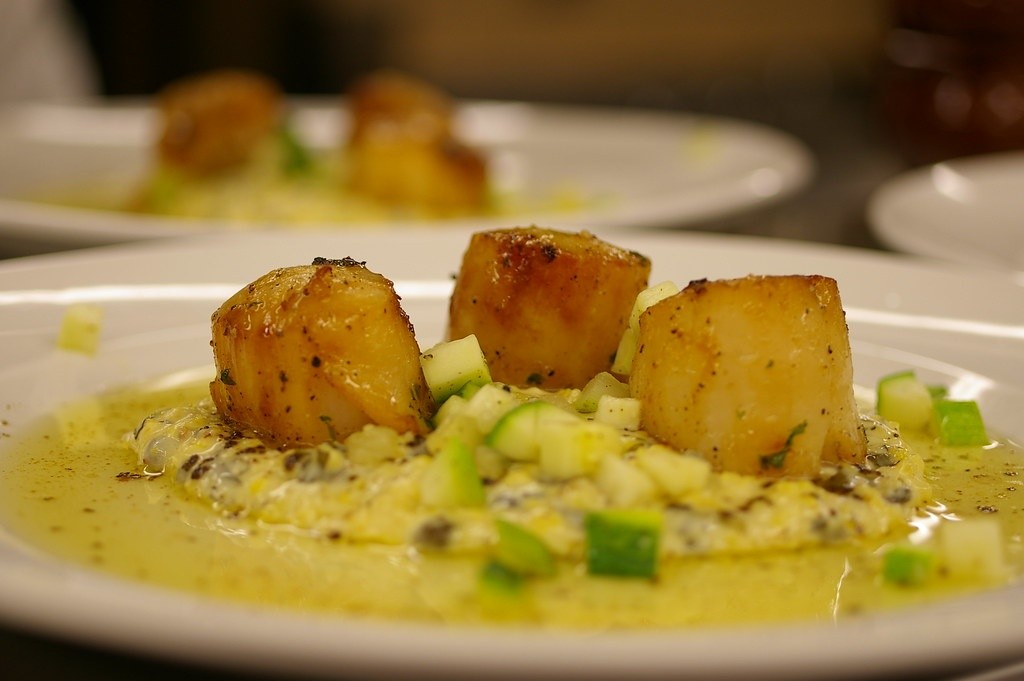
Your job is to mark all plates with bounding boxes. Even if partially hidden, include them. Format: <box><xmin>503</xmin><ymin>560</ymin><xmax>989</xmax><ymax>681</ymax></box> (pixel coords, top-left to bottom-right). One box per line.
<box><xmin>1</xmin><ymin>99</ymin><xmax>813</xmax><ymax>233</ymax></box>
<box><xmin>865</xmin><ymin>152</ymin><xmax>1023</xmax><ymax>269</ymax></box>
<box><xmin>1</xmin><ymin>230</ymin><xmax>1024</xmax><ymax>681</ymax></box>
